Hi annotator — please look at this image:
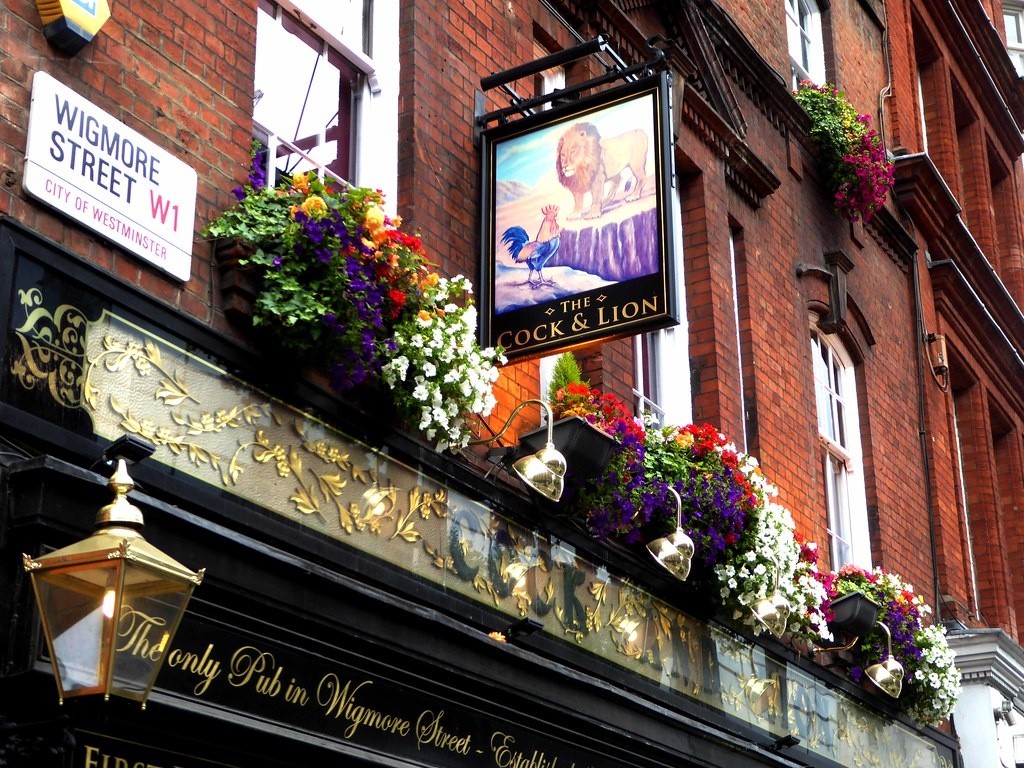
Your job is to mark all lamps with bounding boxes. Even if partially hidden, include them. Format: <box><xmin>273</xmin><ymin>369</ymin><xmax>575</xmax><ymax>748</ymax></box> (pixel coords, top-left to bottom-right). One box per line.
<box><xmin>468</xmin><ymin>399</ymin><xmax>568</xmax><ymax>504</ymax></box>
<box><xmin>865</xmin><ymin>619</ymin><xmax>905</xmax><ymax>700</ymax></box>
<box><xmin>18</xmin><ymin>456</ymin><xmax>206</xmax><ymax>717</ymax></box>
<box><xmin>645</xmin><ymin>485</ymin><xmax>696</xmax><ymax>584</ymax></box>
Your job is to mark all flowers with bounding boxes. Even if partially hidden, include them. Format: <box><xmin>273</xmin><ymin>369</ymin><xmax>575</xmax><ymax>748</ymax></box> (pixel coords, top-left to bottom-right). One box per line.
<box><xmin>792</xmin><ymin>76</ymin><xmax>896</xmax><ymax>227</ymax></box>
<box><xmin>205</xmin><ymin>138</ymin><xmax>512</xmax><ymax>459</ymax></box>
<box><xmin>542</xmin><ymin>352</ymin><xmax>839</xmax><ymax>656</ymax></box>
<box><xmin>841</xmin><ymin>562</ymin><xmax>964</xmax><ymax>736</ymax></box>
